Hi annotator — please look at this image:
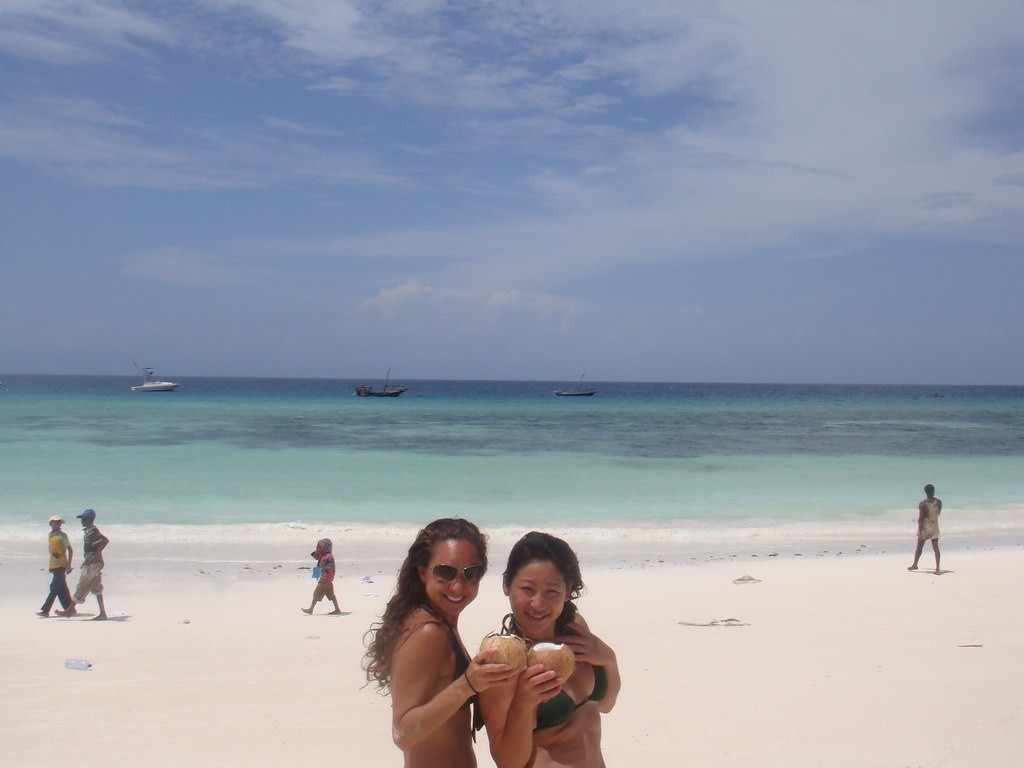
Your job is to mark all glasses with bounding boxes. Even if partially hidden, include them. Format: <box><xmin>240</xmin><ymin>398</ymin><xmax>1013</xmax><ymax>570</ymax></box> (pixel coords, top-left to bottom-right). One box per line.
<box><xmin>425</xmin><ymin>565</ymin><xmax>484</xmax><ymax>584</ymax></box>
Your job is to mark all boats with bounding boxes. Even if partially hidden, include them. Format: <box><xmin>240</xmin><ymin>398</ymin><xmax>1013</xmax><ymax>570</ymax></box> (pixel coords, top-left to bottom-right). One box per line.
<box><xmin>554</xmin><ymin>372</ymin><xmax>596</xmax><ymax>396</ymax></box>
<box><xmin>130</xmin><ymin>361</ymin><xmax>179</xmax><ymax>391</ymax></box>
<box><xmin>353</xmin><ymin>368</ymin><xmax>409</xmax><ymax>397</ymax></box>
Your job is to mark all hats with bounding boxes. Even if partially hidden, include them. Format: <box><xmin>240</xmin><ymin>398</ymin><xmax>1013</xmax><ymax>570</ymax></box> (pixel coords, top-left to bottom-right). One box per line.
<box><xmin>76</xmin><ymin>509</ymin><xmax>96</xmax><ymax>520</ymax></box>
<box><xmin>49</xmin><ymin>517</ymin><xmax>65</xmax><ymax>526</ymax></box>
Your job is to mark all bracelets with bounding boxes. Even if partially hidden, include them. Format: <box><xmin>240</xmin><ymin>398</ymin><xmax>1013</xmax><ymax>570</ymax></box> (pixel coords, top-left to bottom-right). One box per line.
<box><xmin>465</xmin><ymin>672</ymin><xmax>480</xmax><ymax>695</ymax></box>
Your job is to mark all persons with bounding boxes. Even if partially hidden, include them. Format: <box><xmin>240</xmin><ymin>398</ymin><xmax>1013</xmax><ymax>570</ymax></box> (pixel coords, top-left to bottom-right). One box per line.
<box><xmin>55</xmin><ymin>509</ymin><xmax>109</xmax><ymax>620</ymax></box>
<box><xmin>478</xmin><ymin>532</ymin><xmax>621</xmax><ymax>768</ymax></box>
<box><xmin>301</xmin><ymin>538</ymin><xmax>341</xmax><ymax>615</ymax></box>
<box><xmin>36</xmin><ymin>516</ymin><xmax>73</xmax><ymax>617</ymax></box>
<box><xmin>359</xmin><ymin>518</ymin><xmax>518</xmax><ymax>768</ymax></box>
<box><xmin>907</xmin><ymin>484</ymin><xmax>943</xmax><ymax>575</ymax></box>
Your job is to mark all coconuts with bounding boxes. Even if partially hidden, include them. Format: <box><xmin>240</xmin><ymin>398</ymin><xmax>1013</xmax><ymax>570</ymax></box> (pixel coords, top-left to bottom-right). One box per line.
<box><xmin>478</xmin><ymin>631</ymin><xmax>528</xmax><ymax>679</ymax></box>
<box><xmin>525</xmin><ymin>642</ymin><xmax>575</xmax><ymax>686</ymax></box>
